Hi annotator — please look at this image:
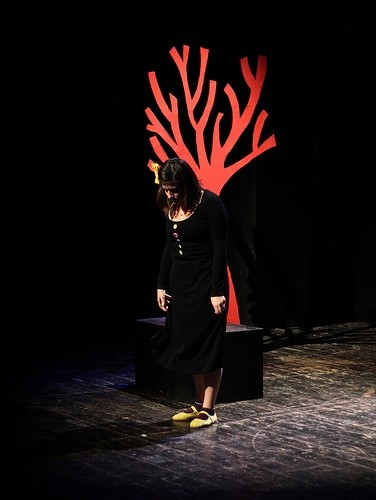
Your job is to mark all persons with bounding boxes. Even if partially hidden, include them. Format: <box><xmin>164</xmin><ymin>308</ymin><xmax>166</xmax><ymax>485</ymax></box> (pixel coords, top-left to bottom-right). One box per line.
<box><xmin>157</xmin><ymin>157</ymin><xmax>232</xmax><ymax>430</ymax></box>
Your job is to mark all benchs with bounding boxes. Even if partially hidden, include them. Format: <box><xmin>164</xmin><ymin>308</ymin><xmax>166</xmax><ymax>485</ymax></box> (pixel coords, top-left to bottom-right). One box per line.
<box><xmin>135</xmin><ymin>313</ymin><xmax>264</xmax><ymax>405</ymax></box>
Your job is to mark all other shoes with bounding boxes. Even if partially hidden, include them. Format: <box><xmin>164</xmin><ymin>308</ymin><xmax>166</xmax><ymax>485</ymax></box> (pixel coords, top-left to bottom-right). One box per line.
<box><xmin>190</xmin><ymin>410</ymin><xmax>218</xmax><ymax>429</ymax></box>
<box><xmin>172</xmin><ymin>405</ymin><xmax>201</xmax><ymax>421</ymax></box>
